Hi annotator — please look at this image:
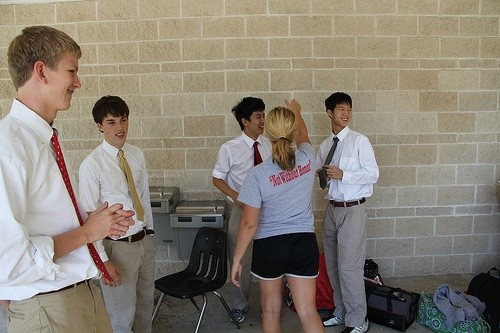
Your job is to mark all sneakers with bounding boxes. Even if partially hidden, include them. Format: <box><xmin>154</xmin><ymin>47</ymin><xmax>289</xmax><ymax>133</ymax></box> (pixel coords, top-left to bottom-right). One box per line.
<box><xmin>230</xmin><ymin>306</ymin><xmax>247</xmax><ymax>322</ymax></box>
<box><xmin>350</xmin><ymin>319</ymin><xmax>369</xmax><ymax>333</ymax></box>
<box><xmin>322</xmin><ymin>317</ymin><xmax>346</xmax><ymax>326</ymax></box>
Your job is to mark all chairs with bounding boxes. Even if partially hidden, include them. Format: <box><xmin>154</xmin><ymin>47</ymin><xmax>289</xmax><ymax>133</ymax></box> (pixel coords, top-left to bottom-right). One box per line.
<box><xmin>151</xmin><ymin>227</ymin><xmax>240</xmax><ymax>333</ymax></box>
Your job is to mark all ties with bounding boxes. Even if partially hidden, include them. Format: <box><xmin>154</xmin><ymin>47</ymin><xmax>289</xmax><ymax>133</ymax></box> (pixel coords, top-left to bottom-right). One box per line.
<box><xmin>318</xmin><ymin>137</ymin><xmax>339</xmax><ymax>190</ymax></box>
<box><xmin>118</xmin><ymin>149</ymin><xmax>145</xmax><ymax>222</ymax></box>
<box><xmin>51</xmin><ymin>131</ymin><xmax>113</xmax><ymax>282</ymax></box>
<box><xmin>253</xmin><ymin>142</ymin><xmax>263</xmax><ymax>166</ymax></box>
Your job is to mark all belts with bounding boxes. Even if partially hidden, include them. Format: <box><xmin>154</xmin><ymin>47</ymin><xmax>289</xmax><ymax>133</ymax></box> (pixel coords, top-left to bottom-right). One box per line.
<box><xmin>329</xmin><ymin>197</ymin><xmax>366</xmax><ymax>207</ymax></box>
<box><xmin>35</xmin><ymin>278</ymin><xmax>92</xmax><ymax>296</ymax></box>
<box><xmin>104</xmin><ymin>227</ymin><xmax>155</xmax><ymax>244</ymax></box>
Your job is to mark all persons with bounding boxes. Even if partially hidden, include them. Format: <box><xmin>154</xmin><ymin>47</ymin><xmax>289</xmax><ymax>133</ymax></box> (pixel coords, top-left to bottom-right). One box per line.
<box><xmin>231</xmin><ymin>99</ymin><xmax>326</xmax><ymax>333</ymax></box>
<box><xmin>0</xmin><ymin>25</ymin><xmax>135</xmax><ymax>333</ymax></box>
<box><xmin>213</xmin><ymin>96</ymin><xmax>274</xmax><ymax>321</ymax></box>
<box><xmin>314</xmin><ymin>93</ymin><xmax>379</xmax><ymax>333</ymax></box>
<box><xmin>78</xmin><ymin>96</ymin><xmax>154</xmax><ymax>333</ymax></box>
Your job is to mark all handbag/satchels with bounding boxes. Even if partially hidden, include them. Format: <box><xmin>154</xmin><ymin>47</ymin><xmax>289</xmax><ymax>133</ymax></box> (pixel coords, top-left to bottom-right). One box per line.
<box><xmin>363</xmin><ymin>279</ymin><xmax>420</xmax><ymax>332</ymax></box>
<box><xmin>283</xmin><ymin>251</ymin><xmax>336</xmax><ymax>313</ymax></box>
<box><xmin>416</xmin><ymin>290</ymin><xmax>491</xmax><ymax>333</ymax></box>
<box><xmin>464</xmin><ymin>267</ymin><xmax>500</xmax><ymax>333</ymax></box>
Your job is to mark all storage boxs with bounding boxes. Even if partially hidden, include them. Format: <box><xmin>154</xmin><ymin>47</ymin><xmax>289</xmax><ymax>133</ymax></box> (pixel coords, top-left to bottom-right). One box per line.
<box><xmin>148</xmin><ymin>187</ymin><xmax>228</xmax><ymax>260</ymax></box>
